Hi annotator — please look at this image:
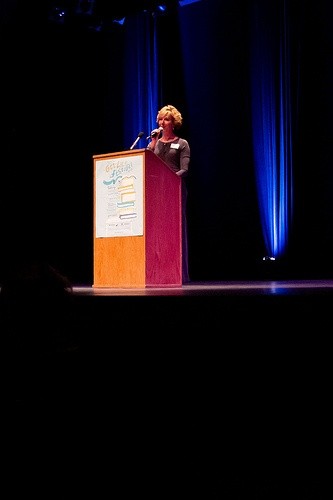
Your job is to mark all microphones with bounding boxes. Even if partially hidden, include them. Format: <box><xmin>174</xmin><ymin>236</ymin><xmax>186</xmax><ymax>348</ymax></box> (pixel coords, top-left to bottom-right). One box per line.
<box><xmin>151</xmin><ymin>127</ymin><xmax>163</xmax><ymax>138</ymax></box>
<box><xmin>130</xmin><ymin>132</ymin><xmax>145</xmax><ymax>149</ymax></box>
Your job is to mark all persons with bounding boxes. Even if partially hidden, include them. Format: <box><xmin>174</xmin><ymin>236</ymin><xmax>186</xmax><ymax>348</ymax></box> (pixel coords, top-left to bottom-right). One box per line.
<box><xmin>147</xmin><ymin>106</ymin><xmax>191</xmax><ymax>177</ymax></box>
